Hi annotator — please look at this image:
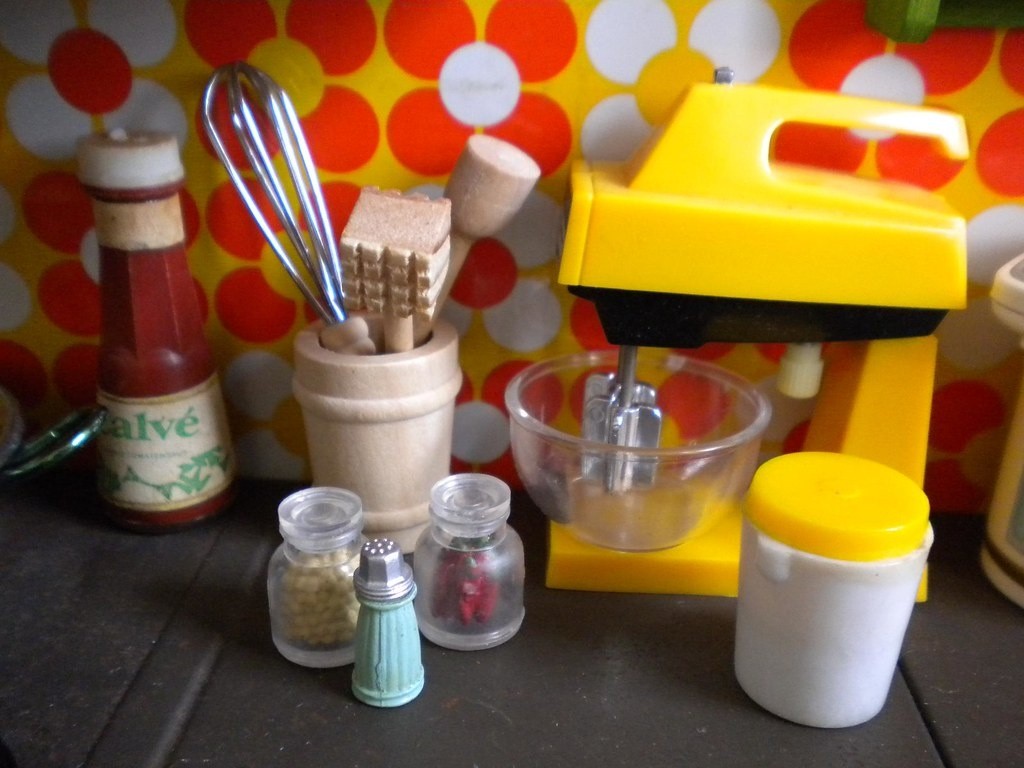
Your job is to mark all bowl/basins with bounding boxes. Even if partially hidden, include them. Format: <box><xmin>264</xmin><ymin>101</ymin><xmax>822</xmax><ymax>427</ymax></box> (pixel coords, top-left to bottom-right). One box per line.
<box><xmin>501</xmin><ymin>347</ymin><xmax>773</xmax><ymax>551</ymax></box>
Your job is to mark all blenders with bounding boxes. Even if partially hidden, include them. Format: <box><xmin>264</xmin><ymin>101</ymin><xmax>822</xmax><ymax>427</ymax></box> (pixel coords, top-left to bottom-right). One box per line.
<box><xmin>544</xmin><ymin>81</ymin><xmax>968</xmax><ymax>602</ymax></box>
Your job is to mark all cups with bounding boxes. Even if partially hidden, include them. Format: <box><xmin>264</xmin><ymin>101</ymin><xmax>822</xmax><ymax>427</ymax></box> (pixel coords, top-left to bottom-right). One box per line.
<box><xmin>291</xmin><ymin>318</ymin><xmax>463</xmax><ymax>556</ymax></box>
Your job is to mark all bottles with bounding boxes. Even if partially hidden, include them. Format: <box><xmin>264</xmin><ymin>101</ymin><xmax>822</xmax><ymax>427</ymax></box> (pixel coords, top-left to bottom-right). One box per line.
<box><xmin>412</xmin><ymin>473</ymin><xmax>528</xmax><ymax>650</ymax></box>
<box><xmin>81</xmin><ymin>131</ymin><xmax>239</xmax><ymax>528</ymax></box>
<box><xmin>268</xmin><ymin>484</ymin><xmax>375</xmax><ymax>670</ymax></box>
<box><xmin>352</xmin><ymin>538</ymin><xmax>424</xmax><ymax>707</ymax></box>
<box><xmin>733</xmin><ymin>449</ymin><xmax>935</xmax><ymax>729</ymax></box>
